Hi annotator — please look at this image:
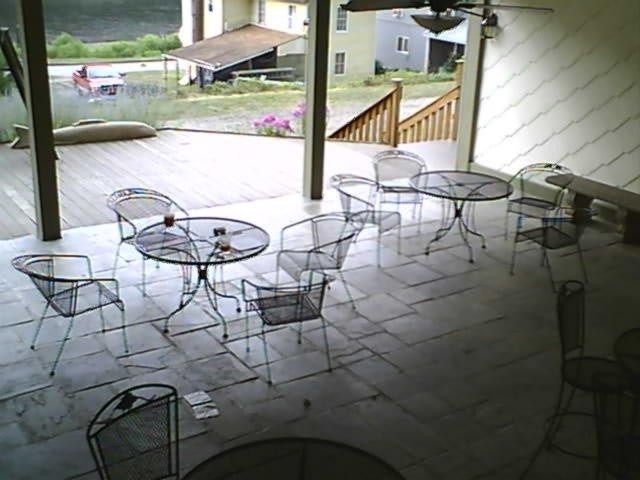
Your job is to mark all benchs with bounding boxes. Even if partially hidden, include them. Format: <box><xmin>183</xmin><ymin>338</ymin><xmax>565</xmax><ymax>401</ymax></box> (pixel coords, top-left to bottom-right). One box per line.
<box><xmin>545</xmin><ymin>171</ymin><xmax>639</xmax><ymax>244</ymax></box>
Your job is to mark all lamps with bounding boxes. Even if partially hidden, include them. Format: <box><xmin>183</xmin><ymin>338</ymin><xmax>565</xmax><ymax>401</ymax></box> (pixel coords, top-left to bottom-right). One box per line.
<box><xmin>411</xmin><ymin>13</ymin><xmax>467</xmax><ymax>34</ymax></box>
<box><xmin>481</xmin><ymin>14</ymin><xmax>498</xmax><ymax>39</ymax></box>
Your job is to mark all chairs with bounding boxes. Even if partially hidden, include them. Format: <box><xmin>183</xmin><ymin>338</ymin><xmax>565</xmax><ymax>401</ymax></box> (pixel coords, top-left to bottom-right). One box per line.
<box><xmin>590</xmin><ymin>370</ymin><xmax>638</xmax><ymax>477</ymax></box>
<box><xmin>274</xmin><ymin>211</ymin><xmax>364</xmax><ymax>314</ymax></box>
<box><xmin>11</xmin><ymin>253</ymin><xmax>129</xmax><ymax>376</ymax></box>
<box><xmin>525</xmin><ymin>279</ymin><xmax>635</xmax><ymax>478</ymax></box>
<box><xmin>84</xmin><ymin>383</ymin><xmax>179</xmax><ymax>478</ymax></box>
<box><xmin>511</xmin><ymin>206</ymin><xmax>591</xmax><ymax>291</ymax></box>
<box><xmin>241</xmin><ymin>268</ymin><xmax>336</xmax><ymax>386</ymax></box>
<box><xmin>371</xmin><ymin>151</ymin><xmax>430</xmax><ymax>233</ymax></box>
<box><xmin>505</xmin><ymin>162</ymin><xmax>572</xmax><ymax>241</ymax></box>
<box><xmin>330</xmin><ymin>172</ymin><xmax>403</xmax><ymax>268</ymax></box>
<box><xmin>106</xmin><ymin>188</ymin><xmax>190</xmax><ymax>297</ymax></box>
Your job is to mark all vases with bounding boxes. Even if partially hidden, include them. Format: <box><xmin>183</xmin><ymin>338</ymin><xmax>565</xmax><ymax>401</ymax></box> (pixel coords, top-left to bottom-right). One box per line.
<box><xmin>302</xmin><ymin>115</ymin><xmax>306</xmax><ymax>136</ymax></box>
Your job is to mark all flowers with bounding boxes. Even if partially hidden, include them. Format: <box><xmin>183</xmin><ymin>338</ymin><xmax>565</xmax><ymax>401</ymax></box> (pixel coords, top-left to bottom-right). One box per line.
<box><xmin>252</xmin><ymin>115</ymin><xmax>294</xmax><ymax>137</ymax></box>
<box><xmin>292</xmin><ymin>101</ymin><xmax>331</xmax><ymax>117</ymax></box>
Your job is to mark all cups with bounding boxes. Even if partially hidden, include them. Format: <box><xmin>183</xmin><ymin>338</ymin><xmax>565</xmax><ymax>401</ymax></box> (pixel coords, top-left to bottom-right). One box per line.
<box><xmin>218</xmin><ymin>235</ymin><xmax>230</xmax><ymax>252</ymax></box>
<box><xmin>164</xmin><ymin>211</ymin><xmax>174</xmax><ymax>228</ymax></box>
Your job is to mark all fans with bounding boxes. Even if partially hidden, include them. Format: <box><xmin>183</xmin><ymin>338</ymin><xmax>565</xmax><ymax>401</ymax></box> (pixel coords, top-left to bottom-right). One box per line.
<box><xmin>340</xmin><ymin>0</ymin><xmax>555</xmax><ymax>17</ymax></box>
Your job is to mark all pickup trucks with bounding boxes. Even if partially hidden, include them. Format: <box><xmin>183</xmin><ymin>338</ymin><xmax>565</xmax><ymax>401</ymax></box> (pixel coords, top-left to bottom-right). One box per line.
<box><xmin>71</xmin><ymin>60</ymin><xmax>127</xmax><ymax>105</ymax></box>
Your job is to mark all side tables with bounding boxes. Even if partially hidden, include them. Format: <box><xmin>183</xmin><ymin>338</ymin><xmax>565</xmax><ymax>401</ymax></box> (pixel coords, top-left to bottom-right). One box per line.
<box><xmin>408</xmin><ymin>168</ymin><xmax>514</xmax><ymax>264</ymax></box>
<box><xmin>614</xmin><ymin>328</ymin><xmax>638</xmax><ymax>381</ymax></box>
<box><xmin>135</xmin><ymin>215</ymin><xmax>270</xmax><ymax>341</ymax></box>
<box><xmin>180</xmin><ymin>438</ymin><xmax>405</xmax><ymax>479</ymax></box>
